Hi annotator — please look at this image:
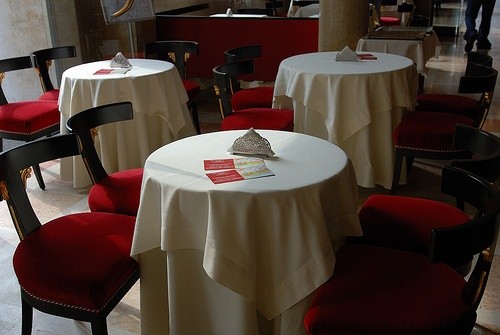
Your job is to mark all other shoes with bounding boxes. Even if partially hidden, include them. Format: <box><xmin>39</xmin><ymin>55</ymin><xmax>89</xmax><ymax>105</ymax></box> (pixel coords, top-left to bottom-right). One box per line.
<box><xmin>476</xmin><ymin>41</ymin><xmax>491</xmax><ymax>48</ymax></box>
<box><xmin>465</xmin><ymin>29</ymin><xmax>477</xmax><ymax>52</ymax></box>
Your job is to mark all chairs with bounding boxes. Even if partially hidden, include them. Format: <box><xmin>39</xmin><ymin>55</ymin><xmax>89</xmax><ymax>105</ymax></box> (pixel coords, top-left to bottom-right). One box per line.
<box><xmin>448</xmin><ymin>121</ymin><xmax>500</xmax><ymax>211</ymax></box>
<box><xmin>300</xmin><ymin>164</ymin><xmax>500</xmax><ymax>335</ymax></box>
<box><xmin>30</xmin><ymin>45</ymin><xmax>77</xmax><ymax>100</ymax></box>
<box><xmin>0</xmin><ymin>132</ymin><xmax>142</xmax><ymax>335</ymax></box>
<box><xmin>65</xmin><ymin>101</ymin><xmax>144</xmax><ymax>215</ymax></box>
<box><xmin>415</xmin><ymin>51</ymin><xmax>493</xmax><ymax>119</ymax></box>
<box><xmin>359</xmin><ymin>165</ymin><xmax>500</xmax><ymax>255</ymax></box>
<box><xmin>389</xmin><ymin>62</ymin><xmax>498</xmax><ymax>211</ymax></box>
<box><xmin>0</xmin><ymin>55</ymin><xmax>60</xmax><ymax>190</ymax></box>
<box><xmin>213</xmin><ymin>59</ymin><xmax>294</xmax><ymax>131</ymax></box>
<box><xmin>223</xmin><ymin>45</ymin><xmax>274</xmax><ymax>112</ymax></box>
<box><xmin>142</xmin><ymin>40</ymin><xmax>202</xmax><ymax>135</ymax></box>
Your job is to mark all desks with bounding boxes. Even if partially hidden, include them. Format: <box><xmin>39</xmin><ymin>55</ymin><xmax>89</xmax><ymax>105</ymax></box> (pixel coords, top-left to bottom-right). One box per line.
<box><xmin>355</xmin><ymin>25</ymin><xmax>441</xmax><ymax>93</ymax></box>
<box><xmin>57</xmin><ymin>59</ymin><xmax>197</xmax><ymax>189</ymax></box>
<box><xmin>271</xmin><ymin>50</ymin><xmax>418</xmax><ymax>190</ymax></box>
<box><xmin>129</xmin><ymin>131</ymin><xmax>364</xmax><ymax>335</ymax></box>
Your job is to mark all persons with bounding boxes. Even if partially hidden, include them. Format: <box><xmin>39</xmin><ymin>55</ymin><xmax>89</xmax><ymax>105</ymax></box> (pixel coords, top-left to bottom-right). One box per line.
<box><xmin>463</xmin><ymin>0</ymin><xmax>500</xmax><ymax>52</ymax></box>
